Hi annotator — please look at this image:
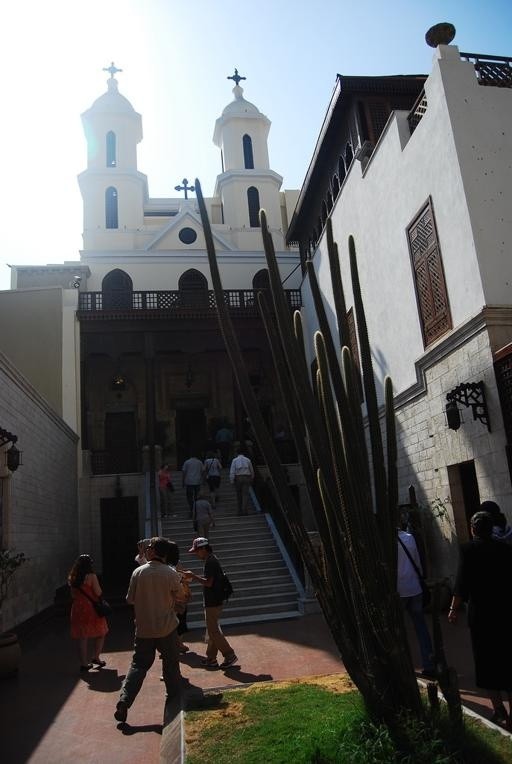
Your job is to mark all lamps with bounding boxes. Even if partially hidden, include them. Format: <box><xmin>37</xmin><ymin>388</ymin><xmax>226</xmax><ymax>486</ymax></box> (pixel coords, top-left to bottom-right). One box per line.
<box><xmin>111</xmin><ymin>365</ymin><xmax>129</xmax><ymax>386</ymax></box>
<box><xmin>445</xmin><ymin>381</ymin><xmax>491</xmax><ymax>436</ymax></box>
<box><xmin>0</xmin><ymin>425</ymin><xmax>25</xmax><ymax>474</ymax></box>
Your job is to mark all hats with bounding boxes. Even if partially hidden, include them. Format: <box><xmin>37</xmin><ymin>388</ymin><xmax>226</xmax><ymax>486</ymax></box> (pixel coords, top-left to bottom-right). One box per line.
<box><xmin>189</xmin><ymin>537</ymin><xmax>209</xmax><ymax>552</ymax></box>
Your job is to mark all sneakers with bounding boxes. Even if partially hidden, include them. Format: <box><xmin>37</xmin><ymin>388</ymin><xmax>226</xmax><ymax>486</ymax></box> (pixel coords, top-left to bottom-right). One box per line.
<box><xmin>114</xmin><ymin>701</ymin><xmax>127</xmax><ymax>722</ymax></box>
<box><xmin>220</xmin><ymin>655</ymin><xmax>238</xmax><ymax>668</ymax></box>
<box><xmin>200</xmin><ymin>659</ymin><xmax>218</xmax><ymax>666</ymax></box>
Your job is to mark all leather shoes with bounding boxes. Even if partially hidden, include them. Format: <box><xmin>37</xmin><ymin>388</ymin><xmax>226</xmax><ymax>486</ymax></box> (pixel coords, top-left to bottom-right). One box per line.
<box><xmin>91</xmin><ymin>659</ymin><xmax>106</xmax><ymax>666</ymax></box>
<box><xmin>80</xmin><ymin>664</ymin><xmax>94</xmax><ymax>671</ymax></box>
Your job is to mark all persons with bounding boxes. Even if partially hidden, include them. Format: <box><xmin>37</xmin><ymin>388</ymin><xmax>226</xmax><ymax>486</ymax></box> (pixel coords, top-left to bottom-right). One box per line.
<box><xmin>229</xmin><ymin>448</ymin><xmax>255</xmax><ymax>516</ymax></box>
<box><xmin>202</xmin><ymin>450</ymin><xmax>223</xmax><ymax>509</ymax></box>
<box><xmin>397</xmin><ymin>520</ymin><xmax>434</xmax><ymax>679</ymax></box>
<box><xmin>181</xmin><ymin>449</ymin><xmax>207</xmax><ymax>518</ymax></box>
<box><xmin>480</xmin><ymin>500</ymin><xmax>512</xmax><ymax>545</ymax></box>
<box><xmin>114</xmin><ymin>539</ymin><xmax>205</xmax><ymax>725</ymax></box>
<box><xmin>215</xmin><ymin>423</ymin><xmax>233</xmax><ymax>468</ymax></box>
<box><xmin>192</xmin><ymin>489</ymin><xmax>216</xmax><ymax>538</ymax></box>
<box><xmin>67</xmin><ymin>553</ymin><xmax>110</xmax><ymax>671</ymax></box>
<box><xmin>158</xmin><ymin>462</ymin><xmax>177</xmax><ymax>517</ymax></box>
<box><xmin>181</xmin><ymin>538</ymin><xmax>242</xmax><ymax>669</ymax></box>
<box><xmin>131</xmin><ymin>540</ymin><xmax>191</xmax><ymax>657</ymax></box>
<box><xmin>447</xmin><ymin>511</ymin><xmax>512</xmax><ymax>733</ymax></box>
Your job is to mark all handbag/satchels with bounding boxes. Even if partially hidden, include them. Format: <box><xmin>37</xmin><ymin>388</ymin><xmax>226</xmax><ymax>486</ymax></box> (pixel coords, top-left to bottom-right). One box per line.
<box><xmin>222</xmin><ymin>574</ymin><xmax>233</xmax><ymax>600</ymax></box>
<box><xmin>94</xmin><ymin>597</ymin><xmax>111</xmax><ymax>615</ymax></box>
<box><xmin>167</xmin><ymin>481</ymin><xmax>172</xmax><ymax>493</ymax></box>
<box><xmin>203</xmin><ymin>472</ymin><xmax>207</xmax><ymax>486</ymax></box>
<box><xmin>193</xmin><ymin>520</ymin><xmax>198</xmax><ymax>531</ymax></box>
<box><xmin>425</xmin><ymin>588</ymin><xmax>435</xmax><ymax>612</ymax></box>
